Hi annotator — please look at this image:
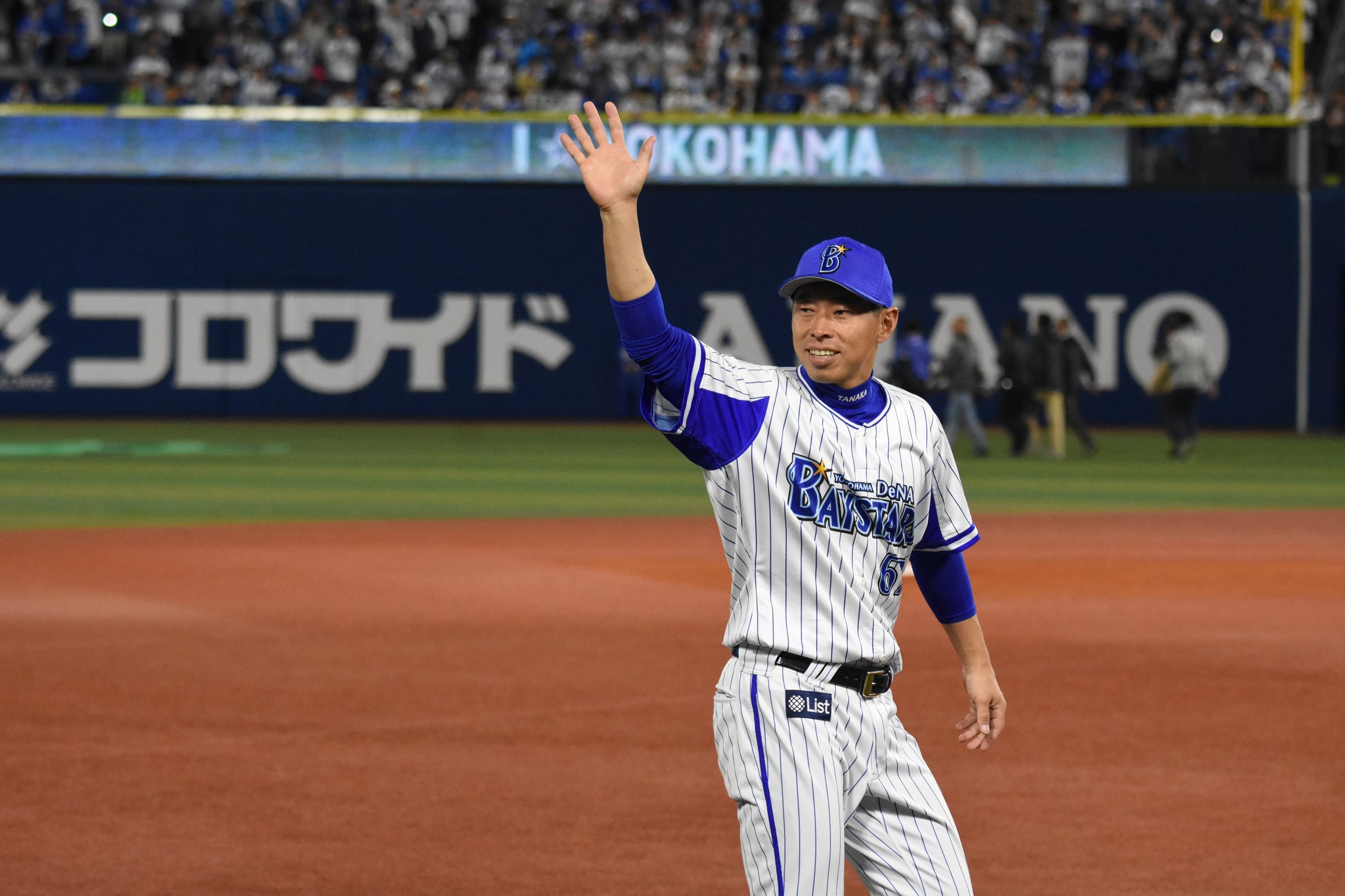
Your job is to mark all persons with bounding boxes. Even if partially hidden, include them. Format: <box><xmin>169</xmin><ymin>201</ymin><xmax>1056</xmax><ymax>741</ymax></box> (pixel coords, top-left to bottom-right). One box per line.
<box><xmin>558</xmin><ymin>95</ymin><xmax>1008</xmax><ymax>896</ymax></box>
<box><xmin>939</xmin><ymin>316</ymin><xmax>992</xmax><ymax>464</ymax></box>
<box><xmin>1150</xmin><ymin>316</ymin><xmax>1216</xmax><ymax>459</ymax></box>
<box><xmin>894</xmin><ymin>314</ymin><xmax>932</xmax><ymax>403</ymax></box>
<box><xmin>999</xmin><ymin>309</ymin><xmax>1094</xmax><ymax>466</ymax></box>
<box><xmin>0</xmin><ymin>0</ymin><xmax>1345</xmax><ymax>186</ymax></box>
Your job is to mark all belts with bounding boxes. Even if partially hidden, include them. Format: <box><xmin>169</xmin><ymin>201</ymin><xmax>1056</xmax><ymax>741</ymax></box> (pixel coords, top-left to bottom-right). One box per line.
<box><xmin>730</xmin><ymin>642</ymin><xmax>893</xmax><ymax>698</ymax></box>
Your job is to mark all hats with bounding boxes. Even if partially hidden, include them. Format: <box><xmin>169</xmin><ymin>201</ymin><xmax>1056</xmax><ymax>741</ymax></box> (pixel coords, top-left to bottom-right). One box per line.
<box><xmin>778</xmin><ymin>238</ymin><xmax>893</xmax><ymax>310</ymax></box>
